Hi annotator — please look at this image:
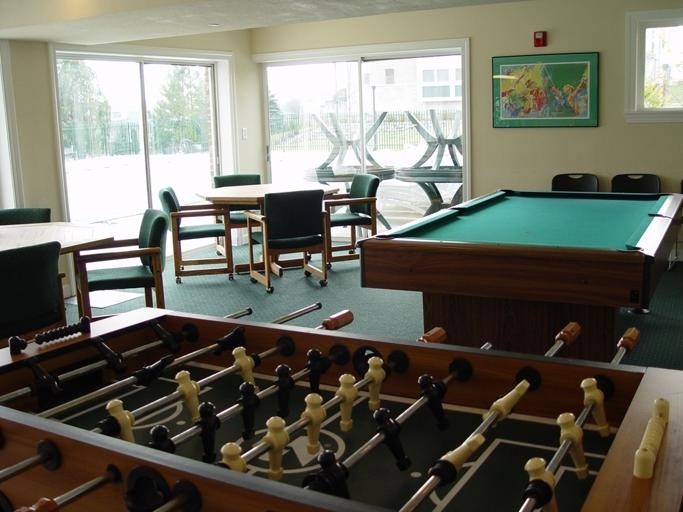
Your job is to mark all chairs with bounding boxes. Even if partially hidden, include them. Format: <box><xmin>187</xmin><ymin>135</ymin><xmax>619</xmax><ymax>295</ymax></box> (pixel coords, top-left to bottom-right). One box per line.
<box><xmin>307</xmin><ymin>173</ymin><xmax>380</xmax><ymax>269</ymax></box>
<box><xmin>610</xmin><ymin>173</ymin><xmax>659</xmax><ymax>194</ymax></box>
<box><xmin>552</xmin><ymin>173</ymin><xmax>598</xmax><ymax>192</ymax></box>
<box><xmin>73</xmin><ymin>207</ymin><xmax>169</xmax><ymax>321</ymax></box>
<box><xmin>159</xmin><ymin>187</ymin><xmax>234</xmax><ymax>284</ymax></box>
<box><xmin>0</xmin><ymin>208</ymin><xmax>51</xmax><ymax>225</ymax></box>
<box><xmin>0</xmin><ymin>241</ymin><xmax>67</xmax><ymax>349</ymax></box>
<box><xmin>244</xmin><ymin>189</ymin><xmax>328</xmax><ymax>294</ymax></box>
<box><xmin>213</xmin><ymin>175</ymin><xmax>261</xmax><ymax>257</ymax></box>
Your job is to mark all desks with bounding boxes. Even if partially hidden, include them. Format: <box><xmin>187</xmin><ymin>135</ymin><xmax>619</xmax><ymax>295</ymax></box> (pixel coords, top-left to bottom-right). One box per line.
<box><xmin>356</xmin><ymin>189</ymin><xmax>683</xmax><ymax>361</ymax></box>
<box><xmin>312</xmin><ymin>112</ymin><xmax>394</xmax><ymax>176</ymax></box>
<box><xmin>397</xmin><ymin>177</ymin><xmax>463</xmax><ymax>217</ymax></box>
<box><xmin>195</xmin><ymin>184</ymin><xmax>340</xmax><ymax>274</ymax></box>
<box><xmin>397</xmin><ymin>109</ymin><xmax>462</xmax><ymax>176</ymax></box>
<box><xmin>318</xmin><ymin>173</ymin><xmax>394</xmax><ymax>230</ymax></box>
<box><xmin>0</xmin><ymin>222</ymin><xmax>114</xmax><ymax>255</ymax></box>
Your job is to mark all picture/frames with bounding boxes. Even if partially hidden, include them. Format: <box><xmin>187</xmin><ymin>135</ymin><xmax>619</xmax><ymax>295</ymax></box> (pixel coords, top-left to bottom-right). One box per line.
<box><xmin>492</xmin><ymin>52</ymin><xmax>599</xmax><ymax>128</ymax></box>
